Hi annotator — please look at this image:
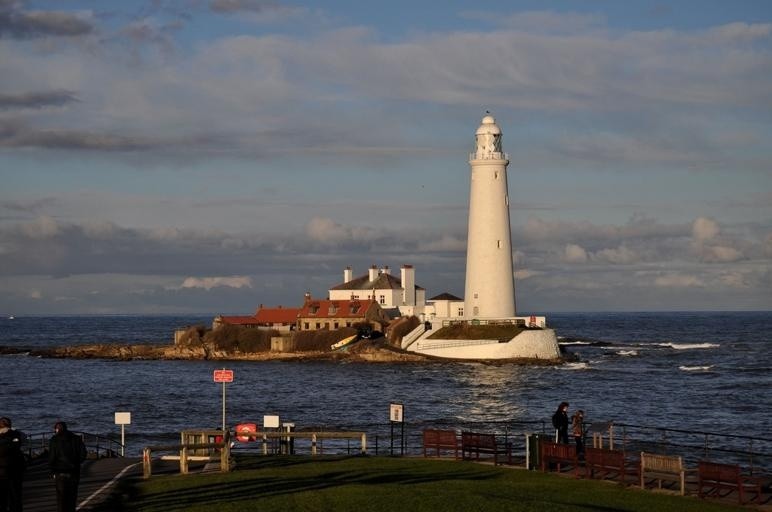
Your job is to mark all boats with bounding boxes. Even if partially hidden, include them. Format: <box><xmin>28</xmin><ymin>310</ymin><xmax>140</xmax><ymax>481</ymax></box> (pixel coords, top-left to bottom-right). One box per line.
<box><xmin>331</xmin><ymin>334</ymin><xmax>359</xmax><ymax>351</ymax></box>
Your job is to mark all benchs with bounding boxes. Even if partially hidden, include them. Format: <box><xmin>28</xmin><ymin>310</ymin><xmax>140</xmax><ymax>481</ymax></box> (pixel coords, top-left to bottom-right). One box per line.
<box><xmin>540</xmin><ymin>442</ymin><xmax>772</xmax><ymax>505</ymax></box>
<box><xmin>422</xmin><ymin>429</ymin><xmax>509</xmax><ymax>467</ymax></box>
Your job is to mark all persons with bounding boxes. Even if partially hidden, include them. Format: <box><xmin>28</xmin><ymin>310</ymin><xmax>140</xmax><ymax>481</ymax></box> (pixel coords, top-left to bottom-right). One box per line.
<box><xmin>552</xmin><ymin>401</ymin><xmax>569</xmax><ymax>444</ymax></box>
<box><xmin>46</xmin><ymin>421</ymin><xmax>88</xmax><ymax>512</ymax></box>
<box><xmin>0</xmin><ymin>417</ymin><xmax>27</xmax><ymax>512</ymax></box>
<box><xmin>569</xmin><ymin>409</ymin><xmax>585</xmax><ymax>453</ymax></box>
